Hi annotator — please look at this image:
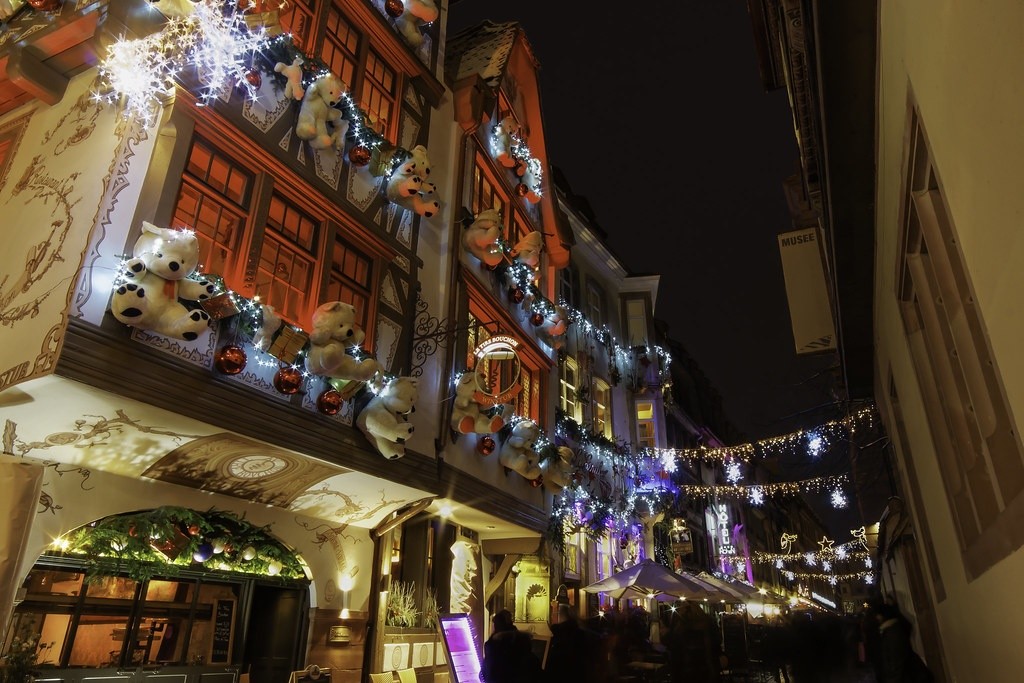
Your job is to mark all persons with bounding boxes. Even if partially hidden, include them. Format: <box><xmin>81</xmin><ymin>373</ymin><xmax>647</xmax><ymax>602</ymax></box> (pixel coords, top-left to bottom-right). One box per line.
<box><xmin>482</xmin><ymin>601</ymin><xmax>933</xmax><ymax>683</ymax></box>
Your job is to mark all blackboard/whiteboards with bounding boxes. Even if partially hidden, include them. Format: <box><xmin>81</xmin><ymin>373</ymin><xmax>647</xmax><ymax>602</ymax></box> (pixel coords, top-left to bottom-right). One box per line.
<box><xmin>206</xmin><ymin>597</ymin><xmax>238</xmax><ymax>666</ymax></box>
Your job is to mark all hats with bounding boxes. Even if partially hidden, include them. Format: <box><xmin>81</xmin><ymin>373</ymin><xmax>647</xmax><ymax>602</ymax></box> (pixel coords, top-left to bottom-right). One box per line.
<box><xmin>872</xmin><ymin>597</ymin><xmax>897</xmax><ymax>618</ymax></box>
<box><xmin>492</xmin><ymin>610</ymin><xmax>512</xmax><ymax>625</ymax></box>
<box><xmin>555</xmin><ymin>604</ymin><xmax>576</xmax><ymax>620</ymax></box>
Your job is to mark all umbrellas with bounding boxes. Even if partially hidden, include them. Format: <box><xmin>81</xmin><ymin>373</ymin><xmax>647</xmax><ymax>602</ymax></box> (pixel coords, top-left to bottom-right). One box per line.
<box><xmin>580</xmin><ymin>558</ymin><xmax>759</xmax><ymax>604</ymax></box>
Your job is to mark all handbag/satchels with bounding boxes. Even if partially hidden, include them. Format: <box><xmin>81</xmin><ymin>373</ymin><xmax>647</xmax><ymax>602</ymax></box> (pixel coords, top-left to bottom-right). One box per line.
<box><xmin>902</xmin><ymin>646</ymin><xmax>934</xmax><ymax>683</ymax></box>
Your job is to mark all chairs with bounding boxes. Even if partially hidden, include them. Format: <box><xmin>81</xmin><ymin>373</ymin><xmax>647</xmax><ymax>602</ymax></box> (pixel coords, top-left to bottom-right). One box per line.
<box><xmin>369</xmin><ymin>671</ymin><xmax>394</xmax><ymax>683</ymax></box>
<box><xmin>396</xmin><ymin>668</ymin><xmax>417</xmax><ymax>683</ymax></box>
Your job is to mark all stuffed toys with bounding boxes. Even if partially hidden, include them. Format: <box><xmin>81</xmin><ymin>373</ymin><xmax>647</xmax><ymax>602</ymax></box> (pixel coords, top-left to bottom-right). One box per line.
<box><xmin>463</xmin><ymin>208</ymin><xmax>504</xmax><ymax>266</ymax></box>
<box><xmin>541</xmin><ymin>447</ymin><xmax>573</xmax><ymax>496</ymax></box>
<box><xmin>274</xmin><ymin>57</ymin><xmax>305</xmax><ymax>101</ymax></box>
<box><xmin>305</xmin><ymin>300</ymin><xmax>377</xmax><ymax>383</ymax></box>
<box><xmin>385</xmin><ymin>145</ymin><xmax>441</xmax><ymax>218</ymax></box>
<box><xmin>112</xmin><ymin>219</ymin><xmax>212</xmax><ymax>342</ymax></box>
<box><xmin>356</xmin><ymin>378</ymin><xmax>419</xmax><ymax>462</ymax></box>
<box><xmin>451</xmin><ymin>372</ymin><xmax>505</xmax><ymax>435</ymax></box>
<box><xmin>500</xmin><ymin>420</ymin><xmax>541</xmax><ymax>480</ymax></box>
<box><xmin>295</xmin><ymin>72</ymin><xmax>349</xmax><ymax>150</ymax></box>
<box><xmin>511</xmin><ymin>229</ymin><xmax>544</xmax><ymax>266</ymax></box>
<box><xmin>541</xmin><ymin>304</ymin><xmax>567</xmax><ymax>336</ymax></box>
<box><xmin>494</xmin><ymin>115</ymin><xmax>520</xmax><ymax>169</ymax></box>
<box><xmin>392</xmin><ymin>1</ymin><xmax>438</xmax><ymax>46</ymax></box>
